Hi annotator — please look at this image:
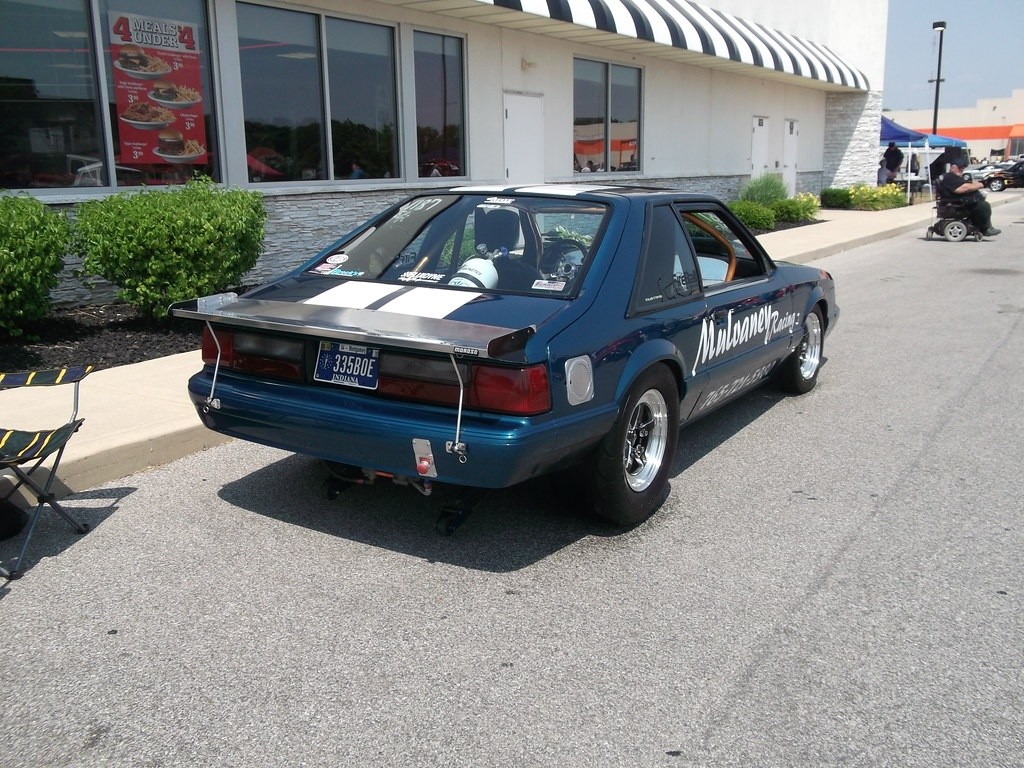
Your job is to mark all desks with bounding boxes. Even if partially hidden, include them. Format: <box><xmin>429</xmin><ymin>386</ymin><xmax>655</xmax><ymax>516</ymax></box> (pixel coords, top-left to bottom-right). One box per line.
<box><xmin>893</xmin><ymin>177</ymin><xmax>927</xmax><ymax>198</ymax></box>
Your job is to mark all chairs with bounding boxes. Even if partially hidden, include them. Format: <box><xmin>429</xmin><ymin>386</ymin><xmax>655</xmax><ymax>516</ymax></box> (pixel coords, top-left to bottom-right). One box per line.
<box><xmin>0</xmin><ymin>365</ymin><xmax>94</xmax><ymax>583</ymax></box>
<box><xmin>481</xmin><ymin>210</ymin><xmax>544</xmax><ymax>290</ymax></box>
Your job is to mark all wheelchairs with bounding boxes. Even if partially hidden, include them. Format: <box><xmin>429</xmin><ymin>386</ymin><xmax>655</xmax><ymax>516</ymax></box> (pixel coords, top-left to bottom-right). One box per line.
<box><xmin>925</xmin><ymin>173</ymin><xmax>985</xmax><ymax>242</ymax></box>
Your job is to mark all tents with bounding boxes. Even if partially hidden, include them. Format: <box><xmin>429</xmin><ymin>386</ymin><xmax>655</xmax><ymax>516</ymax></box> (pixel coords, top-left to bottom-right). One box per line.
<box><xmin>880</xmin><ymin>115</ymin><xmax>966</xmax><ymax>205</ymax></box>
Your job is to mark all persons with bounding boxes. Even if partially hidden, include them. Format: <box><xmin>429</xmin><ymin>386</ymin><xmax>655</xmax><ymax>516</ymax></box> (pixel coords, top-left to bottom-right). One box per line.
<box><xmin>623</xmin><ymin>154</ymin><xmax>638</xmax><ymax>171</ymax></box>
<box><xmin>430</xmin><ymin>166</ymin><xmax>442</xmax><ymax>177</ymax></box>
<box><xmin>884</xmin><ymin>142</ymin><xmax>903</xmax><ymax>173</ymax></box>
<box><xmin>878</xmin><ymin>159</ymin><xmax>896</xmax><ymax>185</ymax></box>
<box><xmin>905</xmin><ymin>154</ymin><xmax>920</xmax><ymax>192</ymax></box>
<box><xmin>586</xmin><ymin>161</ymin><xmax>596</xmax><ymax>172</ymax></box>
<box><xmin>349</xmin><ymin>162</ymin><xmax>367</xmax><ymax>179</ymax></box>
<box><xmin>940</xmin><ymin>159</ymin><xmax>1001</xmax><ymax>236</ymax></box>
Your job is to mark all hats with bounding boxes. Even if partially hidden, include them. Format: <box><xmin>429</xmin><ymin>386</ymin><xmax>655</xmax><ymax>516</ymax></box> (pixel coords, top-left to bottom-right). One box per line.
<box><xmin>951</xmin><ymin>159</ymin><xmax>968</xmax><ymax>168</ymax></box>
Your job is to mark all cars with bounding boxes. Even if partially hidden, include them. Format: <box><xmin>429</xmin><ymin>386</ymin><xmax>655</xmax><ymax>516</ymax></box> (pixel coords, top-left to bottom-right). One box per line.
<box><xmin>959</xmin><ymin>154</ymin><xmax>1024</xmax><ymax>192</ymax></box>
<box><xmin>167</xmin><ymin>181</ymin><xmax>843</xmax><ymax>528</ymax></box>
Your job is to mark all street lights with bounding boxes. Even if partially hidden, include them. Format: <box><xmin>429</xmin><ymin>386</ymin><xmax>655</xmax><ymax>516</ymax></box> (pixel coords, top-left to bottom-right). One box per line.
<box><xmin>926</xmin><ymin>20</ymin><xmax>948</xmax><ymax>150</ymax></box>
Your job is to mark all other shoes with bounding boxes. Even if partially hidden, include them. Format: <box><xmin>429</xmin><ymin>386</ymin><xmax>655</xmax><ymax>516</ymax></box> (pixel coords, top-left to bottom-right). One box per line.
<box><xmin>984</xmin><ymin>227</ymin><xmax>1001</xmax><ymax>236</ymax></box>
